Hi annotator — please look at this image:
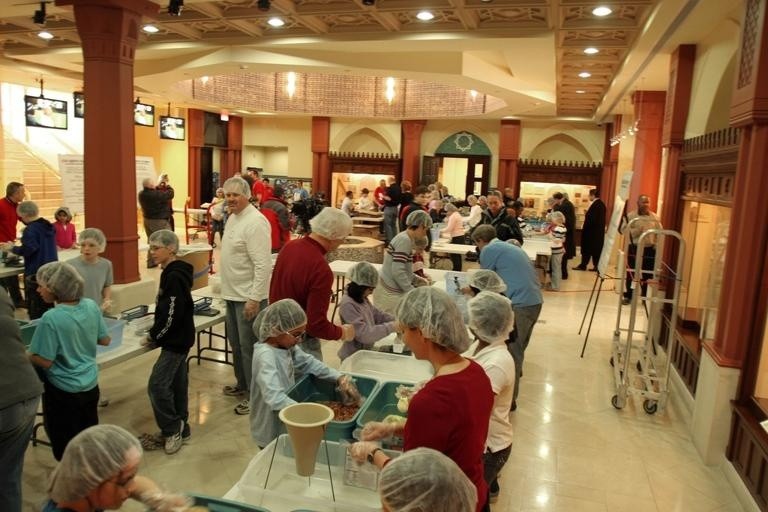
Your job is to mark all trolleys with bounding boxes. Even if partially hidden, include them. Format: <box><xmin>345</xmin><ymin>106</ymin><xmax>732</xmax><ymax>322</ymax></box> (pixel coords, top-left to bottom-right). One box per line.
<box><xmin>609</xmin><ymin>215</ymin><xmax>686</xmax><ymax>415</ymax></box>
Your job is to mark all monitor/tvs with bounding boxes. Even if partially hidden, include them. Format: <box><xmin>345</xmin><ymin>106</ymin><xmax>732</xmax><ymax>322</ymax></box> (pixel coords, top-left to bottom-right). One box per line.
<box><xmin>24</xmin><ymin>94</ymin><xmax>69</xmax><ymax>131</ymax></box>
<box><xmin>73</xmin><ymin>92</ymin><xmax>84</xmax><ymax>118</ymax></box>
<box><xmin>158</xmin><ymin>116</ymin><xmax>185</xmax><ymax>142</ymax></box>
<box><xmin>135</xmin><ymin>103</ymin><xmax>155</xmax><ymax>129</ymax></box>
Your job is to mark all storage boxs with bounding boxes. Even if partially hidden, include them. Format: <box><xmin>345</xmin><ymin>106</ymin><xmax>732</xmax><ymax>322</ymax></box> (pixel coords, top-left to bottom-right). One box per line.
<box><xmin>339</xmin><ymin>350</ymin><xmax>431</xmax><ymax>390</ymax></box>
<box><xmin>357</xmin><ymin>380</ymin><xmax>415</xmax><ymax>431</ymax></box>
<box><xmin>274</xmin><ymin>367</ymin><xmax>378</xmax><ymax>443</ymax></box>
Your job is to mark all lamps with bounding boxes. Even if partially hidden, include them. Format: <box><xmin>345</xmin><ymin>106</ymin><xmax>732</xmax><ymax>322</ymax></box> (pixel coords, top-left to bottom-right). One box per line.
<box><xmin>34</xmin><ymin>2</ymin><xmax>46</xmax><ymax>24</ymax></box>
<box><xmin>363</xmin><ymin>0</ymin><xmax>375</xmax><ymax>5</ymax></box>
<box><xmin>258</xmin><ymin>0</ymin><xmax>271</xmax><ymax>12</ymax></box>
<box><xmin>221</xmin><ymin>112</ymin><xmax>229</xmax><ymax>121</ymax></box>
<box><xmin>168</xmin><ymin>0</ymin><xmax>185</xmax><ymax>16</ymax></box>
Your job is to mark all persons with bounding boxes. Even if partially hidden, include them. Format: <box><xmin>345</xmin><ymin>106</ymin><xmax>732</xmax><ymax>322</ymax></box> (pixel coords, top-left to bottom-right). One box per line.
<box><xmin>137</xmin><ymin>230</ymin><xmax>195</xmax><ymax>454</ymax></box>
<box><xmin>216</xmin><ymin>176</ymin><xmax>273</xmax><ymax>416</ymax></box>
<box><xmin>140</xmin><ymin>172</ymin><xmax>175</xmax><ymax>270</ymax></box>
<box><xmin>472</xmin><ymin>224</ymin><xmax>543</xmax><ymax>410</ymax></box>
<box><xmin>338</xmin><ymin>262</ymin><xmax>402</xmax><ymax>361</ymax></box>
<box><xmin>352</xmin><ymin>285</ymin><xmax>490</xmax><ymax>512</ymax></box>
<box><xmin>249</xmin><ymin>299</ymin><xmax>362</xmax><ymax>449</ymax></box>
<box><xmin>37</xmin><ymin>426</ymin><xmax>195</xmax><ymax>512</ymax></box>
<box><xmin>379</xmin><ymin>447</ymin><xmax>478</xmax><ymax>512</ymax></box>
<box><xmin>204</xmin><ymin>165</ymin><xmax>328</xmax><ymax>255</ymax></box>
<box><xmin>0</xmin><ymin>178</ymin><xmax>113</xmax><ymax>512</ymax></box>
<box><xmin>268</xmin><ymin>207</ymin><xmax>355</xmax><ymax>366</ymax></box>
<box><xmin>618</xmin><ymin>195</ymin><xmax>663</xmax><ymax>305</ymax></box>
<box><xmin>571</xmin><ymin>188</ymin><xmax>607</xmax><ymax>272</ymax></box>
<box><xmin>339</xmin><ymin>178</ymin><xmax>577</xmax><ymax>301</ymax></box>
<box><xmin>462</xmin><ymin>291</ymin><xmax>515</xmax><ymax>502</ymax></box>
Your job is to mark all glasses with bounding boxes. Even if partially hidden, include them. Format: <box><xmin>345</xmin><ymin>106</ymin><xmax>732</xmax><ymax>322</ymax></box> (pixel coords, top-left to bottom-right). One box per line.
<box><xmin>286</xmin><ymin>331</ymin><xmax>305</xmax><ymax>340</ymax></box>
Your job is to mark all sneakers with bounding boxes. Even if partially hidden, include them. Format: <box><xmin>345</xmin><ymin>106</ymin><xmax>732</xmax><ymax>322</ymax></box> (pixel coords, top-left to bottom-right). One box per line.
<box><xmin>224</xmin><ymin>384</ymin><xmax>249</xmax><ymax>414</ymax></box>
<box><xmin>153</xmin><ymin>421</ymin><xmax>190</xmax><ymax>454</ymax></box>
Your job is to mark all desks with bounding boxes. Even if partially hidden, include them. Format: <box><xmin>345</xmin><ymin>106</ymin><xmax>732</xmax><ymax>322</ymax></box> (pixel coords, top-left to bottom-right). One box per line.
<box><xmin>34</xmin><ymin>296</ymin><xmax>228</xmax><ymax>447</ymax></box>
<box><xmin>190</xmin><ymin>432</ymin><xmax>400</xmax><ymax>509</ymax></box>
<box><xmin>2</xmin><ymin>202</ymin><xmax>553</xmax><ymax>353</ymax></box>
